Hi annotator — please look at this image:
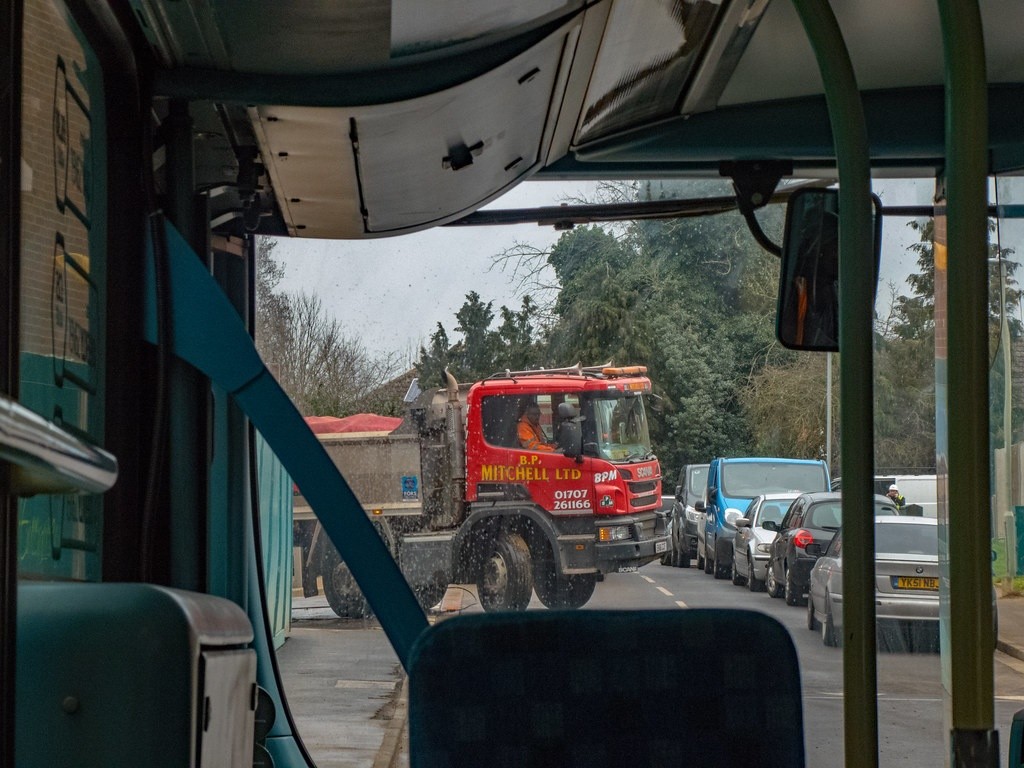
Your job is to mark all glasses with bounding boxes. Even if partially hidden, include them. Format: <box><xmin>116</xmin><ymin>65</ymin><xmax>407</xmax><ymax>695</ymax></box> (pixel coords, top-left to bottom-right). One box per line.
<box><xmin>527</xmin><ymin>413</ymin><xmax>541</xmax><ymax>416</ymax></box>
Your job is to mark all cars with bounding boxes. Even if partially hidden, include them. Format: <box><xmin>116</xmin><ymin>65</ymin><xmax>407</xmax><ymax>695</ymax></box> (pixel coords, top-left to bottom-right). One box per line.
<box><xmin>732</xmin><ymin>493</ymin><xmax>802</xmax><ymax>592</ymax></box>
<box><xmin>804</xmin><ymin>515</ymin><xmax>998</xmax><ymax>654</ymax></box>
<box><xmin>762</xmin><ymin>491</ymin><xmax>903</xmax><ymax>607</ymax></box>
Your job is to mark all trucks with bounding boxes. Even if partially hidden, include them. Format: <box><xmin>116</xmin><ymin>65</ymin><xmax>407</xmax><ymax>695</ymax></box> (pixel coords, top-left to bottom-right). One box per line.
<box><xmin>829</xmin><ymin>473</ymin><xmax>949</xmax><ymax>518</ymax></box>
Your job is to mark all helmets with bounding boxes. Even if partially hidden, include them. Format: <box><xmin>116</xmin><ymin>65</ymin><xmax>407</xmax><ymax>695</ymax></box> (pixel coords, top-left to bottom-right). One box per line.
<box><xmin>888</xmin><ymin>484</ymin><xmax>899</xmax><ymax>491</ymax></box>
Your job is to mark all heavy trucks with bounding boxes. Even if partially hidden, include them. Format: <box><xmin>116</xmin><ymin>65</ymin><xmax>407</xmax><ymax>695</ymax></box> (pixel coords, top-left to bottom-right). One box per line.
<box><xmin>292</xmin><ymin>360</ymin><xmax>675</xmax><ymax>619</ymax></box>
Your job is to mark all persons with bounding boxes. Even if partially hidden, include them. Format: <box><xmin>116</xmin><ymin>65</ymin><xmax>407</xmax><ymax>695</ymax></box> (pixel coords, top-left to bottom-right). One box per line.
<box><xmin>516</xmin><ymin>403</ymin><xmax>559</xmax><ymax>453</ymax></box>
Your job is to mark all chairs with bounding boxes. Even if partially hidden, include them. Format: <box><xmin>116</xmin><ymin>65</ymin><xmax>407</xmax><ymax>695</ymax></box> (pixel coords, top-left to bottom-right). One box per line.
<box><xmin>557</xmin><ymin>401</ymin><xmax>582</xmax><ymax>453</ymax></box>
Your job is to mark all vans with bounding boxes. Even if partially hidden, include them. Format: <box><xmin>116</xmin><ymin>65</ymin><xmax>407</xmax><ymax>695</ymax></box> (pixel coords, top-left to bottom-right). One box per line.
<box><xmin>655</xmin><ymin>463</ymin><xmax>711</xmax><ymax>569</ymax></box>
<box><xmin>694</xmin><ymin>456</ymin><xmax>833</xmax><ymax>580</ymax></box>
